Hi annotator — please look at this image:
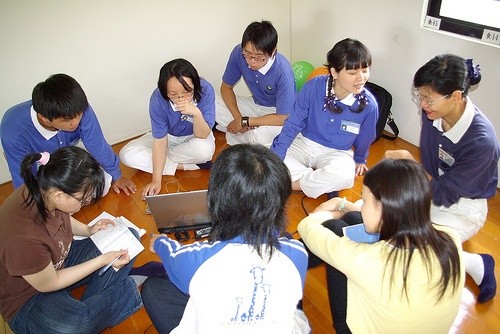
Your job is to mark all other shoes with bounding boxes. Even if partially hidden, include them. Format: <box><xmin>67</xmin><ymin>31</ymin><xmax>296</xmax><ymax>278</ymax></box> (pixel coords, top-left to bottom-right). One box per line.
<box><xmin>128</xmin><ymin>261</ymin><xmax>164</xmax><ymax>277</ymax></box>
<box><xmin>196</xmin><ymin>161</ymin><xmax>214</xmax><ymax>170</ymax></box>
<box><xmin>476</xmin><ymin>253</ymin><xmax>497</xmax><ymax>303</ymax></box>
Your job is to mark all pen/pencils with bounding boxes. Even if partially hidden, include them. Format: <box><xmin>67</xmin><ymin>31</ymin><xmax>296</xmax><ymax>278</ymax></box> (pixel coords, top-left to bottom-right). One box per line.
<box><xmin>98</xmin><ymin>249</ymin><xmax>128</xmax><ymax>277</ymax></box>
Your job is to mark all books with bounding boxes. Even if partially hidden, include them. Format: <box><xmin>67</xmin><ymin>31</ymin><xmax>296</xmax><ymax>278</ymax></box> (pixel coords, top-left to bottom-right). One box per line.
<box><xmin>73</xmin><ymin>211</ymin><xmax>147</xmax><ymax>272</ymax></box>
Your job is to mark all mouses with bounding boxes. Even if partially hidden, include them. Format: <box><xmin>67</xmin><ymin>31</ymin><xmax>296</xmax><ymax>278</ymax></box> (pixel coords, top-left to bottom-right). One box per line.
<box><xmin>147</xmin><ymin>191</ymin><xmax>154</xmax><ymax>196</ymax></box>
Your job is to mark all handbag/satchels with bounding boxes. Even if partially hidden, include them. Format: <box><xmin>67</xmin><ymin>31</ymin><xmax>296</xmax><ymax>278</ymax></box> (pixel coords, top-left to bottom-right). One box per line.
<box><xmin>355</xmin><ymin>81</ymin><xmax>399</xmax><ymax>145</ymax></box>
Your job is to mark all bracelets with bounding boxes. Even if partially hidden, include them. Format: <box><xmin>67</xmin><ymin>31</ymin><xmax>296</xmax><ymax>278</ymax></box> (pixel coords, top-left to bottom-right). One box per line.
<box><xmin>241</xmin><ymin>117</ymin><xmax>249</xmax><ymax>127</ymax></box>
<box><xmin>338</xmin><ymin>196</ymin><xmax>346</xmax><ymax>211</ymax></box>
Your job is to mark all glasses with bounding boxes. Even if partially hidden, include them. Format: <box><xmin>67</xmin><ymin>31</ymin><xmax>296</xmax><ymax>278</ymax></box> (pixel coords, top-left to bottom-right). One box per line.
<box><xmin>414</xmin><ymin>88</ymin><xmax>464</xmax><ymax>106</ymax></box>
<box><xmin>67</xmin><ymin>191</ymin><xmax>92</xmax><ymax>206</ymax></box>
<box><xmin>170</xmin><ymin>94</ymin><xmax>193</xmax><ymax>99</ymax></box>
<box><xmin>242</xmin><ymin>53</ymin><xmax>267</xmax><ymax>62</ymax></box>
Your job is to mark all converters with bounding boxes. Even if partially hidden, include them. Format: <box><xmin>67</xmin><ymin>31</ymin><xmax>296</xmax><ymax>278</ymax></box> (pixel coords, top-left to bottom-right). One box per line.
<box><xmin>194</xmin><ymin>227</ymin><xmax>212</xmax><ymax>240</ymax></box>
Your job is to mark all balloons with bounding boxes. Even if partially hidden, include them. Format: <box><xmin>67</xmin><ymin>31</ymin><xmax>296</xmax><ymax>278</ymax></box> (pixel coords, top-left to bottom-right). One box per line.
<box><xmin>291</xmin><ymin>61</ymin><xmax>330</xmax><ymax>92</ymax></box>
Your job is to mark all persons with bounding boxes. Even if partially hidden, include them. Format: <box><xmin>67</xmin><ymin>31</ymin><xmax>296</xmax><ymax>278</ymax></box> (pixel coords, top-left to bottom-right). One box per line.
<box><xmin>0</xmin><ymin>74</ymin><xmax>137</xmax><ymax>216</ymax></box>
<box><xmin>215</xmin><ymin>21</ymin><xmax>299</xmax><ymax>149</ymax></box>
<box><xmin>0</xmin><ymin>147</ymin><xmax>168</xmax><ymax>334</ymax></box>
<box><xmin>118</xmin><ymin>58</ymin><xmax>216</xmax><ymax>200</ymax></box>
<box><xmin>270</xmin><ymin>37</ymin><xmax>379</xmax><ymax>199</ymax></box>
<box><xmin>297</xmin><ymin>159</ymin><xmax>465</xmax><ymax>334</ymax></box>
<box><xmin>385</xmin><ymin>54</ymin><xmax>500</xmax><ymax>304</ymax></box>
<box><xmin>140</xmin><ymin>143</ymin><xmax>312</xmax><ymax>334</ymax></box>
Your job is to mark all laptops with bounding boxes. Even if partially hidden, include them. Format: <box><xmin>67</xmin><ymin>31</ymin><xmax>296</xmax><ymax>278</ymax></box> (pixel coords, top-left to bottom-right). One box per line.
<box><xmin>144</xmin><ymin>190</ymin><xmax>212</xmax><ymax>234</ymax></box>
